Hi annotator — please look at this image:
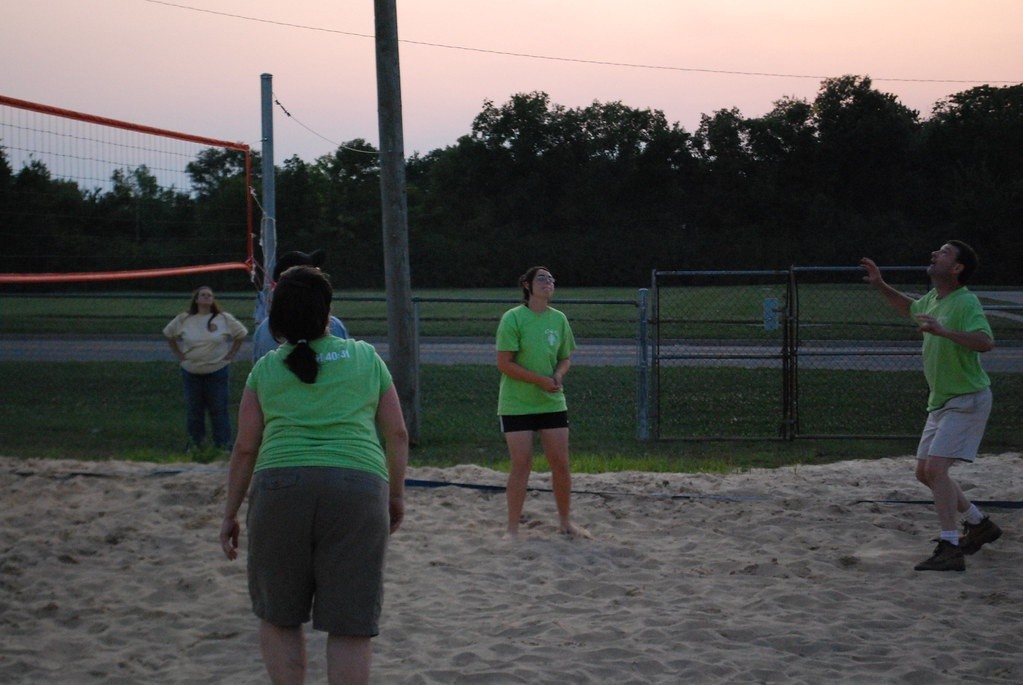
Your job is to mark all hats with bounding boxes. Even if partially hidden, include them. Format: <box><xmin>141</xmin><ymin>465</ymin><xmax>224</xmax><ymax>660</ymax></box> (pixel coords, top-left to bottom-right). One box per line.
<box><xmin>273</xmin><ymin>248</ymin><xmax>324</xmax><ymax>282</ymax></box>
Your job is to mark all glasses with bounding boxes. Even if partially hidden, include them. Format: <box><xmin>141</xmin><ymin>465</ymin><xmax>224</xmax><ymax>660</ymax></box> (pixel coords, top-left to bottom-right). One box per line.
<box><xmin>536</xmin><ymin>276</ymin><xmax>555</xmax><ymax>283</ymax></box>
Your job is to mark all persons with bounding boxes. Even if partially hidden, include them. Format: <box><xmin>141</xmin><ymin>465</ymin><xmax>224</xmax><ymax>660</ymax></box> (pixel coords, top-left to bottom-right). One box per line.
<box><xmin>859</xmin><ymin>240</ymin><xmax>1004</xmax><ymax>573</ymax></box>
<box><xmin>218</xmin><ymin>266</ymin><xmax>409</xmax><ymax>685</ymax></box>
<box><xmin>163</xmin><ymin>287</ymin><xmax>249</xmax><ymax>455</ymax></box>
<box><xmin>496</xmin><ymin>266</ymin><xmax>590</xmax><ymax>539</ymax></box>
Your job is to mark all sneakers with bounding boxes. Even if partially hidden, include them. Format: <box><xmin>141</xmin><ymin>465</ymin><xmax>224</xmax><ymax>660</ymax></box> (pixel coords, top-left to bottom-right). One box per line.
<box><xmin>959</xmin><ymin>516</ymin><xmax>1002</xmax><ymax>555</ymax></box>
<box><xmin>914</xmin><ymin>537</ymin><xmax>966</xmax><ymax>572</ymax></box>
